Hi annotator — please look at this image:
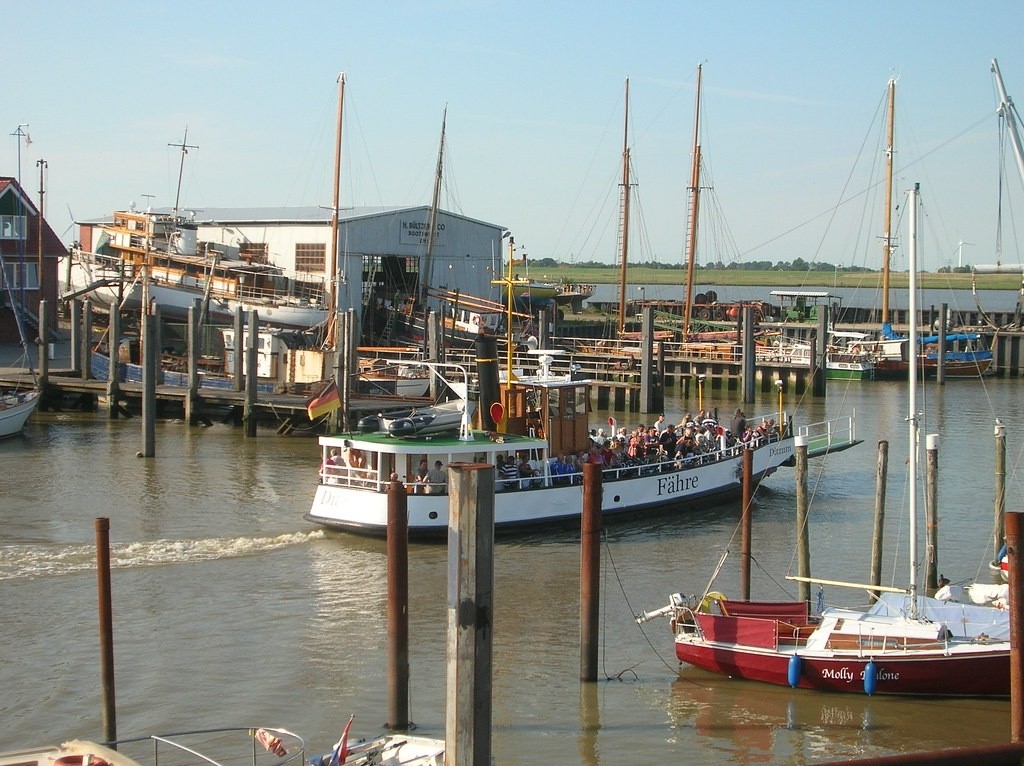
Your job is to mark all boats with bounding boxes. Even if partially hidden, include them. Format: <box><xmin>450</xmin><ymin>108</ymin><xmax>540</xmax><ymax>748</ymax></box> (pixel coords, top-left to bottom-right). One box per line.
<box><xmin>88</xmin><ymin>71</ymin><xmax>430</xmax><ymax>419</ymax></box>
<box><xmin>55</xmin><ymin>124</ymin><xmax>338</xmax><ymax>336</ymax></box>
<box><xmin>355</xmin><ymin>396</ymin><xmax>478</xmax><ymax>439</ymax></box>
<box><xmin>301</xmin><ymin>233</ymin><xmax>869</xmax><ymax>540</ymax></box>
<box><xmin>0</xmin><ymin>712</ymin><xmax>499</xmax><ymax>766</ymax></box>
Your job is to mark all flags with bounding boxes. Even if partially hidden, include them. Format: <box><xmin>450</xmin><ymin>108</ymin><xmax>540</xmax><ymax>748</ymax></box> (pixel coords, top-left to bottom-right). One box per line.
<box><xmin>329</xmin><ymin>720</ymin><xmax>352</xmax><ymax>766</ymax></box>
<box><xmin>303</xmin><ymin>380</ymin><xmax>341</xmax><ymax>421</ymax></box>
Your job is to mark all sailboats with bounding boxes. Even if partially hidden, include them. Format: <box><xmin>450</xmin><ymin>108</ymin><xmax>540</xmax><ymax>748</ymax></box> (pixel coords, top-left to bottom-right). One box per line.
<box><xmin>984</xmin><ymin>57</ymin><xmax>1024</xmax><ymax>582</ymax></box>
<box><xmin>362</xmin><ymin>98</ymin><xmax>539</xmax><ymax>361</ymax></box>
<box><xmin>501</xmin><ymin>59</ymin><xmax>1004</xmax><ymax>382</ymax></box>
<box><xmin>0</xmin><ymin>244</ymin><xmax>47</xmax><ymax>442</ymax></box>
<box><xmin>626</xmin><ymin>181</ymin><xmax>1013</xmax><ymax>703</ymax></box>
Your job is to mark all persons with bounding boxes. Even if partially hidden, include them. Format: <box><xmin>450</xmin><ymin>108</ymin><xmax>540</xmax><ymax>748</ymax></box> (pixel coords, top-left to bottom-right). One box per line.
<box><xmin>414</xmin><ymin>459</ymin><xmax>428</xmax><ymax>493</ymax></box>
<box><xmin>551</xmin><ymin>409</ymin><xmax>776</xmax><ymax>485</ymax></box>
<box><xmin>478</xmin><ymin>457</ymin><xmax>486</xmax><ymax>463</ymax></box>
<box><xmin>496</xmin><ymin>454</ymin><xmax>507</xmax><ymax>469</ymax></box>
<box><xmin>497</xmin><ymin>456</ymin><xmax>520</xmax><ymax>492</ymax></box>
<box><xmin>320</xmin><ymin>449</ymin><xmax>348</xmax><ymax>484</ymax></box>
<box><xmin>519</xmin><ymin>453</ymin><xmax>534</xmax><ymax>489</ymax></box>
<box><xmin>422</xmin><ymin>460</ymin><xmax>446</xmax><ymax>495</ymax></box>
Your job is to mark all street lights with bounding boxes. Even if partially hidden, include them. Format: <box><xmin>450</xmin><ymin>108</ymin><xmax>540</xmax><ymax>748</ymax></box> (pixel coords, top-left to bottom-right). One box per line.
<box><xmin>34</xmin><ymin>158</ymin><xmax>50</xmax><ymax>300</ymax></box>
<box><xmin>636</xmin><ymin>286</ymin><xmax>645</xmax><ymax>311</ymax></box>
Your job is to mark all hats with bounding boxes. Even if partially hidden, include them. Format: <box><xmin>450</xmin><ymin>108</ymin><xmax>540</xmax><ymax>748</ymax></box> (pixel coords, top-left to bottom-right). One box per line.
<box><xmin>660</xmin><ymin>413</ymin><xmax>666</xmax><ymax>416</ymax></box>
<box><xmin>686</xmin><ymin>422</ymin><xmax>696</xmax><ymax>427</ymax></box>
<box><xmin>638</xmin><ymin>424</ymin><xmax>647</xmax><ymax>427</ymax></box>
<box><xmin>435</xmin><ymin>460</ymin><xmax>444</xmax><ymax>466</ymax></box>
<box><xmin>508</xmin><ymin>456</ymin><xmax>516</xmax><ymax>460</ymax></box>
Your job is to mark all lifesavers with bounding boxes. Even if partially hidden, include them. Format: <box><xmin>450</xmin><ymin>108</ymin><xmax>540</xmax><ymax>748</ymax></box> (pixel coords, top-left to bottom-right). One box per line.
<box><xmin>701</xmin><ymin>591</ymin><xmax>728</xmax><ymax>614</ymax></box>
<box><xmin>349</xmin><ymin>451</ymin><xmax>366</xmax><ymax>468</ymax></box>
